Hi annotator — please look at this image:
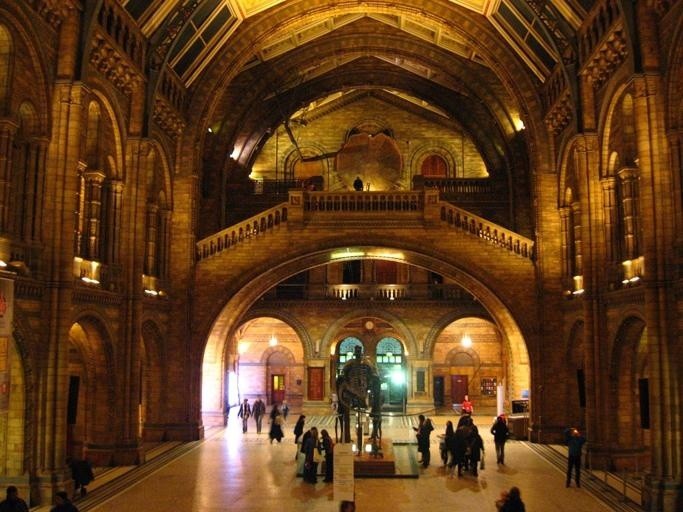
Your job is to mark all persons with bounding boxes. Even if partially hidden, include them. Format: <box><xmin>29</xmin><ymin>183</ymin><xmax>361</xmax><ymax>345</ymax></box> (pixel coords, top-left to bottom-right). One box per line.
<box><xmin>0</xmin><ymin>486</ymin><xmax>29</xmax><ymax>511</ymax></box>
<box><xmin>410</xmin><ymin>394</ymin><xmax>485</xmax><ymax>479</ymax></box>
<box><xmin>339</xmin><ymin>500</ymin><xmax>356</xmax><ymax>512</ymax></box>
<box><xmin>352</xmin><ymin>176</ymin><xmax>362</xmax><ymax>191</ymax></box>
<box><xmin>562</xmin><ymin>427</ymin><xmax>585</xmax><ymax>488</ymax></box>
<box><xmin>236</xmin><ymin>399</ymin><xmax>251</xmax><ymax>434</ymax></box>
<box><xmin>298</xmin><ymin>425</ymin><xmax>336</xmax><ymax>484</ymax></box>
<box><xmin>269</xmin><ymin>405</ymin><xmax>280</xmax><ymax>421</ymax></box>
<box><xmin>293</xmin><ymin>414</ymin><xmax>305</xmax><ymax>461</ymax></box>
<box><xmin>268</xmin><ymin>416</ymin><xmax>283</xmax><ymax>444</ymax></box>
<box><xmin>490</xmin><ymin>416</ymin><xmax>507</xmax><ymax>464</ymax></box>
<box><xmin>48</xmin><ymin>491</ymin><xmax>79</xmax><ymax>511</ymax></box>
<box><xmin>250</xmin><ymin>398</ymin><xmax>265</xmax><ymax>435</ymax></box>
<box><xmin>65</xmin><ymin>457</ymin><xmax>93</xmax><ymax>498</ymax></box>
<box><xmin>493</xmin><ymin>487</ymin><xmax>524</xmax><ymax>512</ymax></box>
<box><xmin>280</xmin><ymin>400</ymin><xmax>287</xmax><ymax>421</ymax></box>
